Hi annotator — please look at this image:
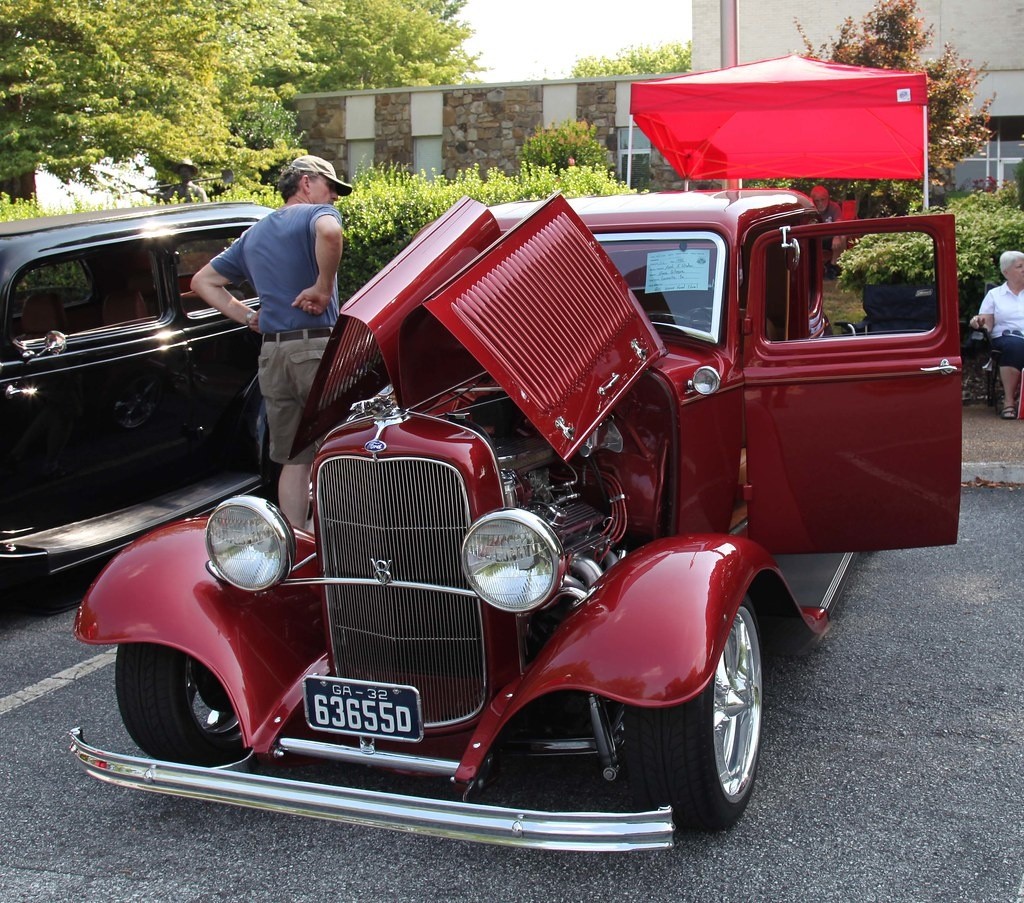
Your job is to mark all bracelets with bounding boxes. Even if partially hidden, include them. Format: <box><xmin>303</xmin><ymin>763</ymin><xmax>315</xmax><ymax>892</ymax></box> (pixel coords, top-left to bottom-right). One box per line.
<box><xmin>246</xmin><ymin>310</ymin><xmax>256</xmax><ymax>324</ymax></box>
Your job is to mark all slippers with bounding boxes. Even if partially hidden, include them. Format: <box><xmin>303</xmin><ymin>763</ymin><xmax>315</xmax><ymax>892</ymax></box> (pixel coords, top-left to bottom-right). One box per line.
<box><xmin>1001</xmin><ymin>406</ymin><xmax>1016</xmax><ymax>418</ymax></box>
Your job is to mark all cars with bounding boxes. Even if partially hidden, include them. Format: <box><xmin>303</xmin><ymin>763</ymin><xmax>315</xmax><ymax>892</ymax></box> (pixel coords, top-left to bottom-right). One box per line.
<box><xmin>66</xmin><ymin>187</ymin><xmax>964</xmax><ymax>851</ymax></box>
<box><xmin>0</xmin><ymin>201</ymin><xmax>275</xmax><ymax>616</ymax></box>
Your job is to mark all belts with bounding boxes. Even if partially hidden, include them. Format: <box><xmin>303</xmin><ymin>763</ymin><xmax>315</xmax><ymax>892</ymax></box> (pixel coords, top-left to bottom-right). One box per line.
<box><xmin>263</xmin><ymin>327</ymin><xmax>331</xmax><ymax>343</ymax></box>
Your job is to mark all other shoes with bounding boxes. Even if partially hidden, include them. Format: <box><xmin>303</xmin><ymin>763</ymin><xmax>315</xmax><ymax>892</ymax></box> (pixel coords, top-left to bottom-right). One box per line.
<box><xmin>826</xmin><ymin>260</ymin><xmax>841</xmax><ymax>280</ymax></box>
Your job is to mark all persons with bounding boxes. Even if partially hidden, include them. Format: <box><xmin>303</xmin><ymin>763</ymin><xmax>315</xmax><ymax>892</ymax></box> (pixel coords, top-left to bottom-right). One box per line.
<box><xmin>809</xmin><ymin>185</ymin><xmax>860</xmax><ymax>268</ymax></box>
<box><xmin>970</xmin><ymin>250</ymin><xmax>1024</xmax><ymax>419</ymax></box>
<box><xmin>188</xmin><ymin>154</ymin><xmax>353</xmax><ymax>531</ymax></box>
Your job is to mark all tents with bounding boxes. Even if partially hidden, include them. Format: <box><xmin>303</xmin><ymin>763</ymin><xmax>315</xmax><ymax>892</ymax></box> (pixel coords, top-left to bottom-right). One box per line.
<box><xmin>626</xmin><ymin>53</ymin><xmax>932</xmax><ymax>213</ymax></box>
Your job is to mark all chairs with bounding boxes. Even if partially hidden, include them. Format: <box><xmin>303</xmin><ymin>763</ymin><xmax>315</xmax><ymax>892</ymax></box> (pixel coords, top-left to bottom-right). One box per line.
<box><xmin>833</xmin><ymin>283</ymin><xmax>940</xmax><ymax>337</ymax></box>
<box><xmin>99</xmin><ymin>283</ymin><xmax>146</xmax><ymax>328</ymax></box>
<box><xmin>21</xmin><ymin>293</ymin><xmax>71</xmax><ymax>338</ymax></box>
<box><xmin>966</xmin><ymin>281</ymin><xmax>1005</xmax><ymax>407</ymax></box>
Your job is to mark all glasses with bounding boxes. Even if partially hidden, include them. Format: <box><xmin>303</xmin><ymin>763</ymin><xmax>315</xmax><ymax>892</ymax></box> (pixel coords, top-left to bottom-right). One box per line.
<box><xmin>1002</xmin><ymin>330</ymin><xmax>1023</xmax><ymax>337</ymax></box>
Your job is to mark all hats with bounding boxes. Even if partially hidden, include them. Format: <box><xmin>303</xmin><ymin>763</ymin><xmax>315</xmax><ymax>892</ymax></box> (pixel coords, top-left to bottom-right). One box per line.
<box><xmin>290</xmin><ymin>154</ymin><xmax>353</xmax><ymax>197</ymax></box>
<box><xmin>174</xmin><ymin>158</ymin><xmax>199</xmax><ymax>176</ymax></box>
